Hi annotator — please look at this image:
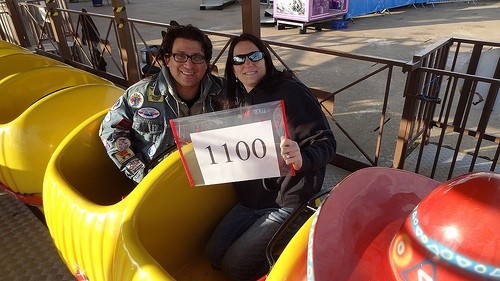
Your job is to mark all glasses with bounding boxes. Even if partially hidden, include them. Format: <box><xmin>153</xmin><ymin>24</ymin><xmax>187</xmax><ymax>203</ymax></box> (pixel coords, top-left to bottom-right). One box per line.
<box><xmin>231</xmin><ymin>50</ymin><xmax>266</xmax><ymax>65</ymax></box>
<box><xmin>165</xmin><ymin>53</ymin><xmax>206</xmax><ymax>64</ymax></box>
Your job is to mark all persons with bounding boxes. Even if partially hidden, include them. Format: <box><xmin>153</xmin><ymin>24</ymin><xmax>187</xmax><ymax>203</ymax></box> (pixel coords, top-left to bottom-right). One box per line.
<box><xmin>99</xmin><ymin>26</ymin><xmax>224</xmax><ymax>184</ymax></box>
<box><xmin>207</xmin><ymin>33</ymin><xmax>337</xmax><ymax>281</ymax></box>
